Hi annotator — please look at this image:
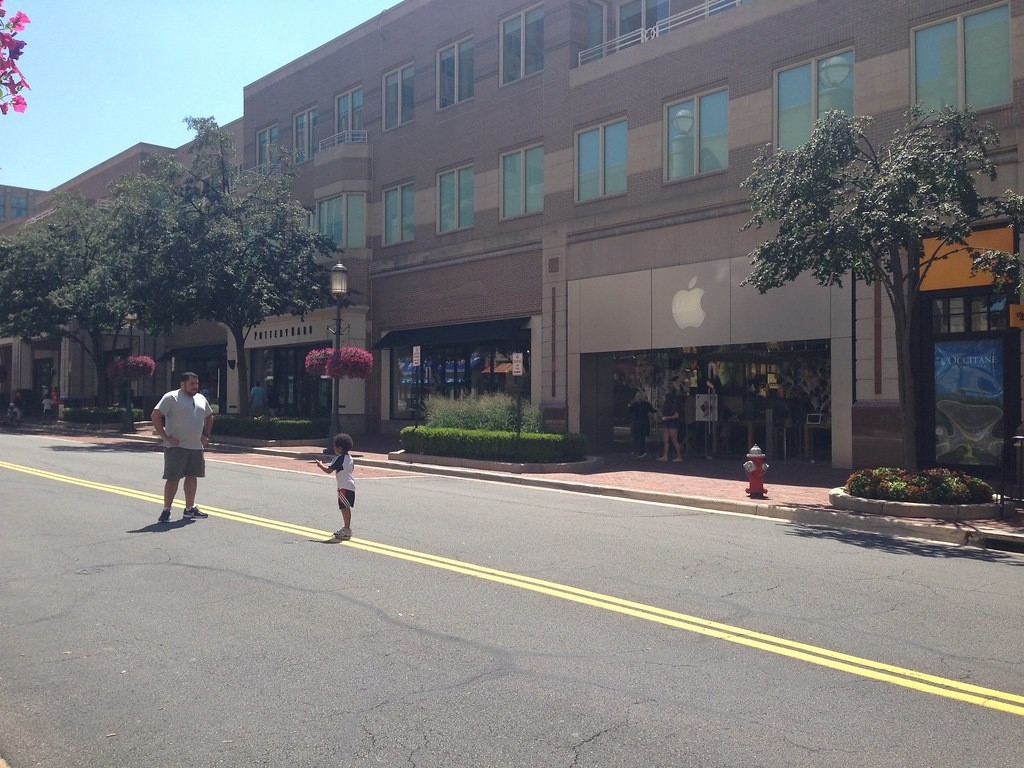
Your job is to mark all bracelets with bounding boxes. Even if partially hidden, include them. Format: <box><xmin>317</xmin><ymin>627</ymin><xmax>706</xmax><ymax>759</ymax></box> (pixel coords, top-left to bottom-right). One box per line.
<box><xmin>204</xmin><ymin>433</ymin><xmax>210</xmax><ymax>438</ymax></box>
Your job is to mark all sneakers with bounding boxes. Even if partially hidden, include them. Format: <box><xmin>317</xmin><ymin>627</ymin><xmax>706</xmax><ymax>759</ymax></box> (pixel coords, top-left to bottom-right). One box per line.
<box><xmin>332</xmin><ymin>526</ymin><xmax>353</xmax><ymax>540</ymax></box>
<box><xmin>158</xmin><ymin>509</ymin><xmax>172</xmax><ymax>523</ymax></box>
<box><xmin>183</xmin><ymin>507</ymin><xmax>208</xmax><ymax>519</ymax></box>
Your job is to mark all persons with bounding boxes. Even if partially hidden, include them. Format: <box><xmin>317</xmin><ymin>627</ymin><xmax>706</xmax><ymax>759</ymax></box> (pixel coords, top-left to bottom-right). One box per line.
<box><xmin>316</xmin><ymin>433</ymin><xmax>355</xmax><ymax>537</ymax></box>
<box><xmin>6</xmin><ymin>385</ymin><xmax>59</xmax><ymax>425</ymax></box>
<box><xmin>151</xmin><ymin>372</ymin><xmax>213</xmax><ymax>522</ymax></box>
<box><xmin>657</xmin><ymin>394</ymin><xmax>684</xmax><ymax>462</ymax></box>
<box><xmin>629</xmin><ymin>391</ymin><xmax>656</xmax><ymax>460</ymax></box>
<box><xmin>250</xmin><ymin>380</ymin><xmax>265</xmax><ymax>416</ymax></box>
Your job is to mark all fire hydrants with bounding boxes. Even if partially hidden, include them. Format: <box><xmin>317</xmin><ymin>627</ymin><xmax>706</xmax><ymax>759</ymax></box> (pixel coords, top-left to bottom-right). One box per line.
<box><xmin>744</xmin><ymin>442</ymin><xmax>770</xmax><ymax>499</ymax></box>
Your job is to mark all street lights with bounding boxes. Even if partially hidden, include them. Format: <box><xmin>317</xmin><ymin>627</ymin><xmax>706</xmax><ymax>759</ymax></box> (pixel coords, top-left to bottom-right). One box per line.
<box><xmin>322</xmin><ymin>259</ymin><xmax>356</xmax><ymax>457</ymax></box>
